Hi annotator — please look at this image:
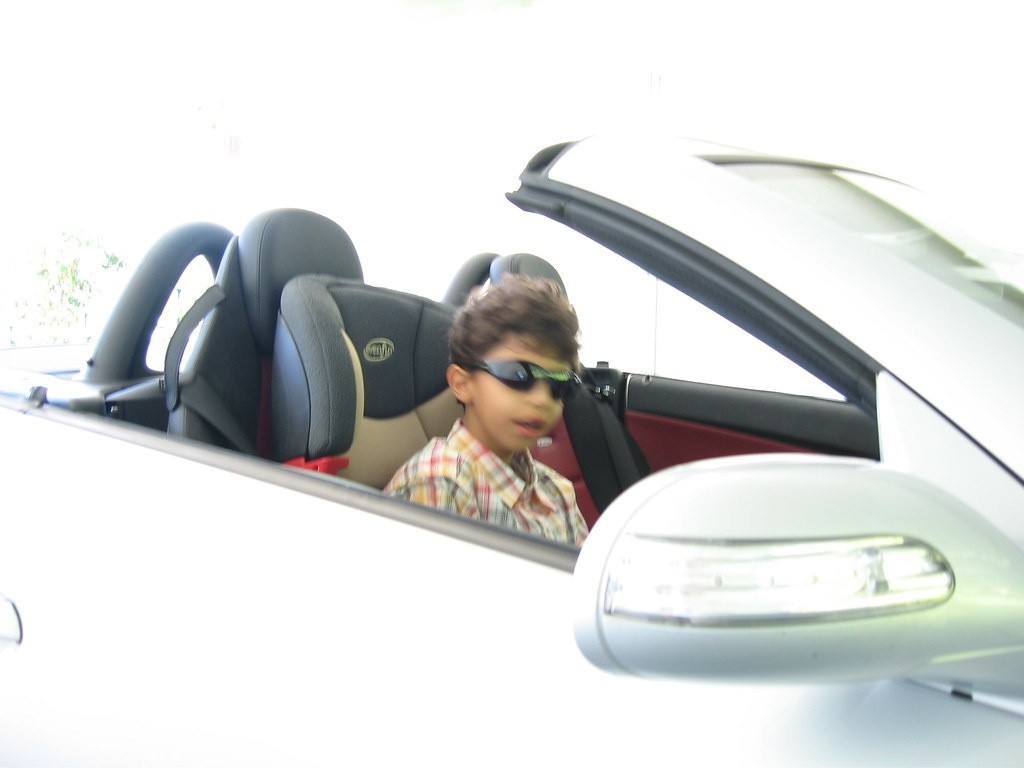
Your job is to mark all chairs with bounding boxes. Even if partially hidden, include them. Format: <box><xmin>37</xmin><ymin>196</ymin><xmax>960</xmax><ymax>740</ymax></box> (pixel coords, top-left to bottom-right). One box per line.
<box><xmin>166</xmin><ymin>206</ymin><xmax>465</xmax><ymax>489</ymax></box>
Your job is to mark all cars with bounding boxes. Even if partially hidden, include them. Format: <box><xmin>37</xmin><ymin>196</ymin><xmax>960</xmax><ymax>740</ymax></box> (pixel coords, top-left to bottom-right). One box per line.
<box><xmin>0</xmin><ymin>131</ymin><xmax>1021</xmax><ymax>768</ymax></box>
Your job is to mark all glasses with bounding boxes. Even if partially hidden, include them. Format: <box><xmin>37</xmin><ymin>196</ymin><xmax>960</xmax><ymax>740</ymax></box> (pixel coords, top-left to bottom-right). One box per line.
<box><xmin>449</xmin><ymin>355</ymin><xmax>583</xmax><ymax>406</ymax></box>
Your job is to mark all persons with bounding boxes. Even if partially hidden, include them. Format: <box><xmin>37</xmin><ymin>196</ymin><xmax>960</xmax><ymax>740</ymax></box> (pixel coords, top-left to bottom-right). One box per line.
<box><xmin>383</xmin><ymin>274</ymin><xmax>591</xmax><ymax>550</ymax></box>
